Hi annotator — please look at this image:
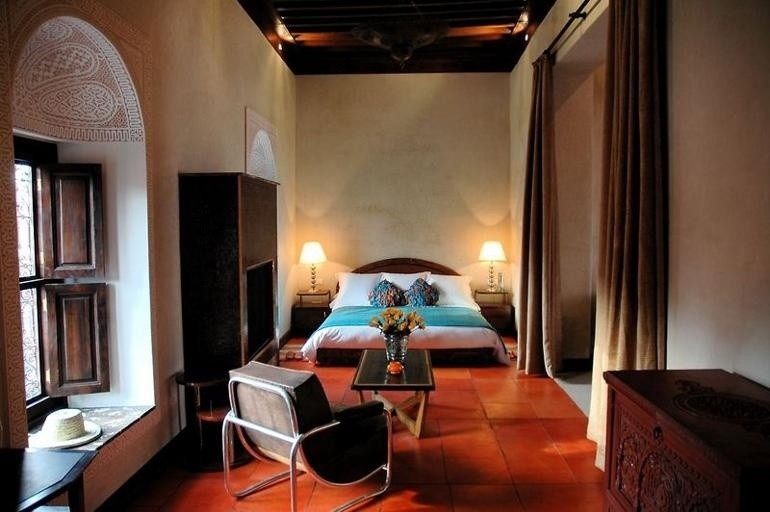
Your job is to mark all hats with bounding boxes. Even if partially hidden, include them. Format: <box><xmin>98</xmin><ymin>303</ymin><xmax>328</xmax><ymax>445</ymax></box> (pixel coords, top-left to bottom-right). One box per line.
<box><xmin>28</xmin><ymin>408</ymin><xmax>102</xmax><ymax>449</ymax></box>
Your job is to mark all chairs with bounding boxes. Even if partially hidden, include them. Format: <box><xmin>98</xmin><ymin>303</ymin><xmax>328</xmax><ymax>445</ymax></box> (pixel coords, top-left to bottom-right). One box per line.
<box><xmin>220</xmin><ymin>358</ymin><xmax>396</xmax><ymax>512</ymax></box>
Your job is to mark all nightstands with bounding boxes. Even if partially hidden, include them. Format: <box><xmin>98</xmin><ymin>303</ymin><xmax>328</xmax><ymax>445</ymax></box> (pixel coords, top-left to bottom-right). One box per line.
<box><xmin>291</xmin><ymin>290</ymin><xmax>334</xmax><ymax>335</ymax></box>
<box><xmin>474</xmin><ymin>289</ymin><xmax>516</xmax><ymax>336</ymax></box>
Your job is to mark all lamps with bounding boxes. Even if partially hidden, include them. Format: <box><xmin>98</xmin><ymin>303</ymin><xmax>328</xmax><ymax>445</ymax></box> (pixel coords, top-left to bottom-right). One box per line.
<box><xmin>476</xmin><ymin>238</ymin><xmax>505</xmax><ymax>292</ymax></box>
<box><xmin>297</xmin><ymin>240</ymin><xmax>329</xmax><ymax>295</ymax></box>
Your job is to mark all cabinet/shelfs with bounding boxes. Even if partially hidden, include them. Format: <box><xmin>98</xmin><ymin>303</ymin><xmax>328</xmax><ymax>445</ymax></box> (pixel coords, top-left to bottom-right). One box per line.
<box><xmin>177</xmin><ymin>171</ymin><xmax>281</xmax><ymax>389</ymax></box>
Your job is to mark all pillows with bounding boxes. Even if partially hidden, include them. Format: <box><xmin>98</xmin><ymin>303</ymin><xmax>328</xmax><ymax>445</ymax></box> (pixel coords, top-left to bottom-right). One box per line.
<box><xmin>432</xmin><ymin>273</ymin><xmax>482</xmax><ymax>313</ymax></box>
<box><xmin>402</xmin><ymin>277</ymin><xmax>441</xmax><ymax>307</ymax></box>
<box><xmin>365</xmin><ymin>277</ymin><xmax>406</xmax><ymax>307</ymax></box>
<box><xmin>382</xmin><ymin>271</ymin><xmax>432</xmax><ymax>292</ymax></box>
<box><xmin>328</xmin><ymin>271</ymin><xmax>381</xmax><ymax>310</ymax></box>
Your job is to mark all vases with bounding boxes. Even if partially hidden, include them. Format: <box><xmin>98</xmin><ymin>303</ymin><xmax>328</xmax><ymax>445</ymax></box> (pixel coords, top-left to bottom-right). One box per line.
<box><xmin>381</xmin><ymin>332</ymin><xmax>411</xmax><ymax>364</ymax></box>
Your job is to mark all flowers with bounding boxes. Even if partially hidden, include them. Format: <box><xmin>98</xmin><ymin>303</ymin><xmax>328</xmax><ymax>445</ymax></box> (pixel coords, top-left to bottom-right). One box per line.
<box><xmin>367</xmin><ymin>305</ymin><xmax>426</xmax><ymax>355</ymax></box>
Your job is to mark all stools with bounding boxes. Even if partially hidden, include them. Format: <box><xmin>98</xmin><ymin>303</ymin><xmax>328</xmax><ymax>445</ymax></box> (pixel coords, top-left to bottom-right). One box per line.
<box><xmin>175</xmin><ymin>369</ymin><xmax>228</xmax><ymax>455</ymax></box>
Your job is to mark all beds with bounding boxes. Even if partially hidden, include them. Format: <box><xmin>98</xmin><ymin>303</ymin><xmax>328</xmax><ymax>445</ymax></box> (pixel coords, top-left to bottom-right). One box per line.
<box><xmin>296</xmin><ymin>255</ymin><xmax>514</xmax><ymax>370</ymax></box>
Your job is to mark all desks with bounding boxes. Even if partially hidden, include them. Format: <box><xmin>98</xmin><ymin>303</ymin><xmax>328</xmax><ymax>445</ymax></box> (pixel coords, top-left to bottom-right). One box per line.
<box><xmin>350</xmin><ymin>346</ymin><xmax>436</xmax><ymax>439</ymax></box>
<box><xmin>1</xmin><ymin>445</ymin><xmax>101</xmax><ymax>512</ymax></box>
<box><xmin>595</xmin><ymin>361</ymin><xmax>770</xmax><ymax>511</ymax></box>
